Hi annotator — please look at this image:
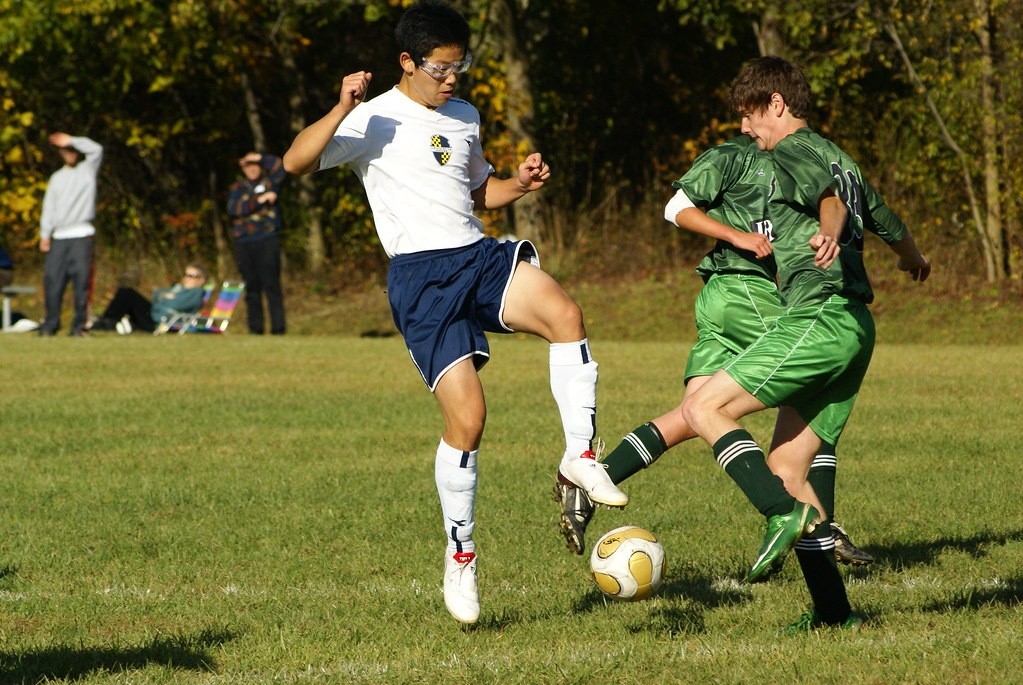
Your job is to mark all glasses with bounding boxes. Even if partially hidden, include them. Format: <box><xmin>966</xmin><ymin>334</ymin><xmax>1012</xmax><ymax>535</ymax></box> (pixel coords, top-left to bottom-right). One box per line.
<box><xmin>419</xmin><ymin>48</ymin><xmax>473</xmax><ymax>82</ymax></box>
<box><xmin>183</xmin><ymin>273</ymin><xmax>204</xmax><ymax>279</ymax></box>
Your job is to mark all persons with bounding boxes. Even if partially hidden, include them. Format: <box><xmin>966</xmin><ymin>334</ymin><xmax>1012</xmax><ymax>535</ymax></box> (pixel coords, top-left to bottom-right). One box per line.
<box><xmin>284</xmin><ymin>0</ymin><xmax>630</xmax><ymax>625</ymax></box>
<box><xmin>82</xmin><ymin>261</ymin><xmax>209</xmax><ymax>335</ymax></box>
<box><xmin>554</xmin><ymin>130</ymin><xmax>877</xmax><ymax>567</ymax></box>
<box><xmin>682</xmin><ymin>59</ymin><xmax>932</xmax><ymax>638</ymax></box>
<box><xmin>0</xmin><ymin>246</ymin><xmax>15</xmax><ymax>290</ymax></box>
<box><xmin>225</xmin><ymin>152</ymin><xmax>286</xmax><ymax>337</ymax></box>
<box><xmin>39</xmin><ymin>132</ymin><xmax>104</xmax><ymax>337</ymax></box>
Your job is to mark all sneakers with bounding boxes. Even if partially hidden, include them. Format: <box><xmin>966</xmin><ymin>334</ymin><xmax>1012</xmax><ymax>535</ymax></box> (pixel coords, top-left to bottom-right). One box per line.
<box><xmin>776</xmin><ymin>610</ymin><xmax>864</xmax><ymax>639</ymax></box>
<box><xmin>545</xmin><ymin>472</ymin><xmax>596</xmax><ymax>557</ymax></box>
<box><xmin>746</xmin><ymin>500</ymin><xmax>823</xmax><ymax>584</ymax></box>
<box><xmin>829</xmin><ymin>522</ymin><xmax>874</xmax><ymax>566</ymax></box>
<box><xmin>443</xmin><ymin>545</ymin><xmax>481</xmax><ymax>624</ymax></box>
<box><xmin>555</xmin><ymin>436</ymin><xmax>629</xmax><ymax>510</ymax></box>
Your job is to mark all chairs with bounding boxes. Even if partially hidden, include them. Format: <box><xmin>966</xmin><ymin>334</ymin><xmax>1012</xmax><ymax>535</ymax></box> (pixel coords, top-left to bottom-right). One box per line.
<box><xmin>154</xmin><ymin>282</ymin><xmax>216</xmax><ymax>331</ymax></box>
<box><xmin>160</xmin><ymin>280</ymin><xmax>244</xmax><ymax>337</ymax></box>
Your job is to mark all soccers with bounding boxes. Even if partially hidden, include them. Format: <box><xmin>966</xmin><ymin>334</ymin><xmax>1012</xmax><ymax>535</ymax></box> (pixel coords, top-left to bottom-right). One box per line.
<box><xmin>589</xmin><ymin>525</ymin><xmax>669</xmax><ymax>603</ymax></box>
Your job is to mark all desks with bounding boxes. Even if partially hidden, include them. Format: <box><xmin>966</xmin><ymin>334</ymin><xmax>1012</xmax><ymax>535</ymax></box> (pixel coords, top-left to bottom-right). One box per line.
<box><xmin>0</xmin><ymin>286</ymin><xmax>38</xmax><ymax>329</ymax></box>
